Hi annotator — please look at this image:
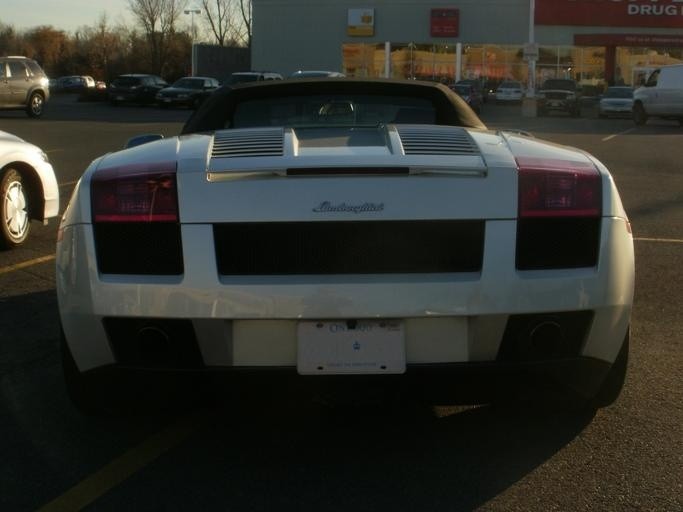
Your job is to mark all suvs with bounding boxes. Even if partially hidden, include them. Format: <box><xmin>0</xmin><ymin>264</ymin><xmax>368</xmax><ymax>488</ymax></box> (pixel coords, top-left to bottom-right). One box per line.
<box><xmin>0</xmin><ymin>55</ymin><xmax>51</xmax><ymax>117</ymax></box>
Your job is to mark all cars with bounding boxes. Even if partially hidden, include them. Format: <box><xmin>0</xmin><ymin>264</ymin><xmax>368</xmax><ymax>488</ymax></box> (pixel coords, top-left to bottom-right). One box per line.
<box><xmin>230</xmin><ymin>71</ymin><xmax>282</xmax><ymax>82</ymax></box>
<box><xmin>48</xmin><ymin>76</ymin><xmax>107</xmax><ymax>93</ymax></box>
<box><xmin>291</xmin><ymin>71</ymin><xmax>347</xmax><ymax>79</ymax></box>
<box><xmin>155</xmin><ymin>78</ymin><xmax>222</xmax><ymax>106</ymax></box>
<box><xmin>447</xmin><ymin>64</ymin><xmax>683</xmax><ymax>125</ymax></box>
<box><xmin>109</xmin><ymin>74</ymin><xmax>169</xmax><ymax>105</ymax></box>
<box><xmin>0</xmin><ymin>129</ymin><xmax>60</xmax><ymax>248</ymax></box>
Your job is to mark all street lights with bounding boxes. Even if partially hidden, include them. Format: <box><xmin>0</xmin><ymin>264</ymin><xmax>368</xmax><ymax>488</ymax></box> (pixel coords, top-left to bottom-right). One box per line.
<box><xmin>184</xmin><ymin>9</ymin><xmax>201</xmax><ymax>77</ymax></box>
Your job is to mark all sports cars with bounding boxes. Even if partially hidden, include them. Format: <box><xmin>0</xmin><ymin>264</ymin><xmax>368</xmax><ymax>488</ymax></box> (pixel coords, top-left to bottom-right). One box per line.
<box><xmin>56</xmin><ymin>79</ymin><xmax>636</xmax><ymax>409</ymax></box>
